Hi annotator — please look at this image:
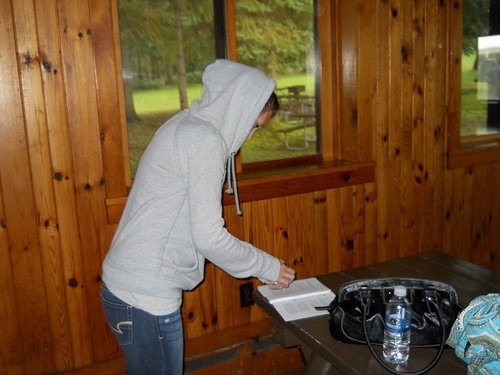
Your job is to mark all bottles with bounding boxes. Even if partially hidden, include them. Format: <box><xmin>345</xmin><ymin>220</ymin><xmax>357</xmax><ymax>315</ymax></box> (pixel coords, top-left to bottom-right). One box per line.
<box><xmin>382</xmin><ymin>286</ymin><xmax>412</xmax><ymax>363</ymax></box>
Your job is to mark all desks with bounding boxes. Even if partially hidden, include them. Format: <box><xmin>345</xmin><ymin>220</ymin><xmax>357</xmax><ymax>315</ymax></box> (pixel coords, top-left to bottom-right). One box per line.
<box><xmin>251</xmin><ymin>249</ymin><xmax>500</xmax><ymax>375</ymax></box>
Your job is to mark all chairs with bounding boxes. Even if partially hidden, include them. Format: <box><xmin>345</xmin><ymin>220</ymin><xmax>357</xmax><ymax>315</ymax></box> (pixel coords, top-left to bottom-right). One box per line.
<box><xmin>274</xmin><ymin>85</ymin><xmax>316</xmax><ymax>150</ymax></box>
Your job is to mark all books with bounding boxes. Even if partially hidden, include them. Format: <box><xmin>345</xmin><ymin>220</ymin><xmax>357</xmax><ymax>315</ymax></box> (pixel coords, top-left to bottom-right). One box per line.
<box><xmin>257</xmin><ymin>277</ymin><xmax>336</xmax><ymax>321</ymax></box>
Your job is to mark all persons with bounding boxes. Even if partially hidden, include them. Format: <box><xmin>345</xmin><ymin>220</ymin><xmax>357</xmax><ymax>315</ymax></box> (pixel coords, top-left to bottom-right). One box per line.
<box><xmin>100</xmin><ymin>59</ymin><xmax>295</xmax><ymax>374</ymax></box>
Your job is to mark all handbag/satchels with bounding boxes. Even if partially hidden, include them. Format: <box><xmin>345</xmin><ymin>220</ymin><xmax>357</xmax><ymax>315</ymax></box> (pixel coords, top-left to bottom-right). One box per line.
<box><xmin>326</xmin><ymin>277</ymin><xmax>467</xmax><ymax>375</ymax></box>
